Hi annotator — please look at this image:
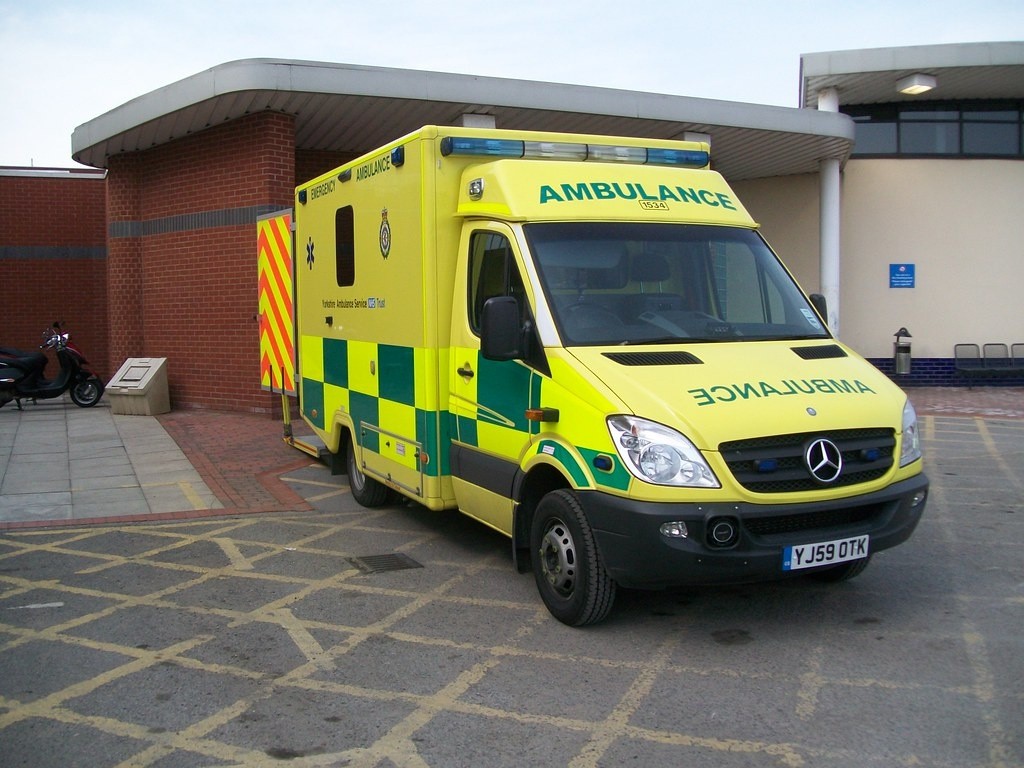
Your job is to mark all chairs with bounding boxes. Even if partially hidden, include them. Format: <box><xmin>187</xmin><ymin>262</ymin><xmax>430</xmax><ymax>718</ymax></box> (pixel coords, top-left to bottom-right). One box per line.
<box><xmin>621</xmin><ymin>257</ymin><xmax>682</xmax><ymax>315</ymax></box>
<box><xmin>954</xmin><ymin>344</ymin><xmax>992</xmax><ymax>391</ymax></box>
<box><xmin>983</xmin><ymin>343</ymin><xmax>1015</xmax><ymax>372</ymax></box>
<box><xmin>1010</xmin><ymin>343</ymin><xmax>1024</xmax><ymax>370</ymax></box>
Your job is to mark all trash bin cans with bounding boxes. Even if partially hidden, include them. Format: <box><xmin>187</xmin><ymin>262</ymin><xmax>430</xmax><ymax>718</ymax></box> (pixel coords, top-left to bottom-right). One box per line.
<box><xmin>892</xmin><ymin>326</ymin><xmax>913</xmax><ymax>376</ymax></box>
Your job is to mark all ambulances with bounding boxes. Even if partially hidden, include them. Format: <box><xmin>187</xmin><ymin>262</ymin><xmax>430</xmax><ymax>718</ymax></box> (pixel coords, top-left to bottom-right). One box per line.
<box><xmin>254</xmin><ymin>123</ymin><xmax>930</xmax><ymax>628</ymax></box>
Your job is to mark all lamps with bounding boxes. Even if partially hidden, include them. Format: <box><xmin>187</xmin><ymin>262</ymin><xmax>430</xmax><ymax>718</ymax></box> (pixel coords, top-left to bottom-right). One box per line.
<box><xmin>896</xmin><ymin>72</ymin><xmax>938</xmax><ymax>96</ymax></box>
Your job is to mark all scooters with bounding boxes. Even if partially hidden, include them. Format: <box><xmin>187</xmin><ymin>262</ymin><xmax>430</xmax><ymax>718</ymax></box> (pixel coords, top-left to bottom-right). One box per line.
<box><xmin>0</xmin><ymin>320</ymin><xmax>104</xmax><ymax>411</ymax></box>
